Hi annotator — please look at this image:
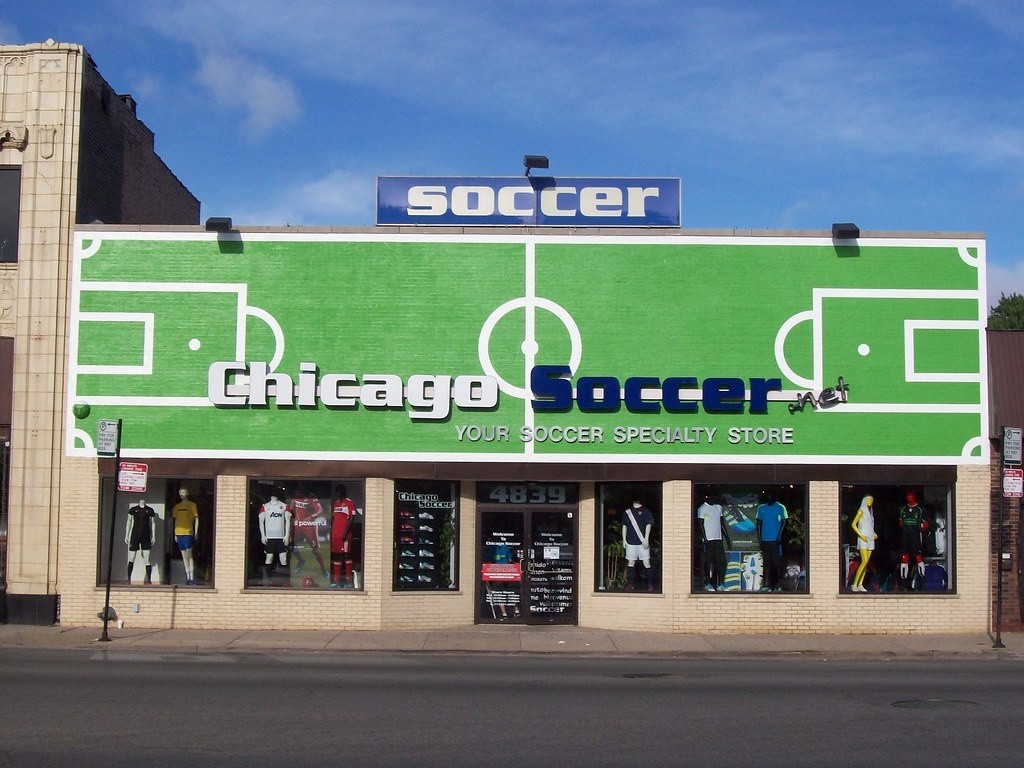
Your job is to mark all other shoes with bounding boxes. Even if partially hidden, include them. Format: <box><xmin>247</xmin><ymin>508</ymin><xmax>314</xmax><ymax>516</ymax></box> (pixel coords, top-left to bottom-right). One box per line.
<box><xmin>500</xmin><ymin>615</ymin><xmax>508</xmax><ymax>620</ymax></box>
<box><xmin>187</xmin><ymin>580</ymin><xmax>195</xmax><ymax>585</ymax></box>
<box><xmin>284</xmin><ymin>581</ymin><xmax>291</xmax><ymax>587</ymax></box>
<box><xmin>144</xmin><ymin>581</ymin><xmax>152</xmax><ymax>585</ymax></box>
<box><xmin>268</xmin><ymin>579</ymin><xmax>273</xmax><ymax>586</ymax></box>
<box><xmin>329</xmin><ymin>582</ymin><xmax>340</xmax><ymax>588</ymax></box>
<box><xmin>344</xmin><ymin>583</ymin><xmax>352</xmax><ymax>589</ymax></box>
<box><xmin>123</xmin><ymin>580</ymin><xmax>131</xmax><ymax>585</ymax></box>
<box><xmin>513</xmin><ymin>611</ymin><xmax>521</xmax><ymax>620</ymax></box>
<box><xmin>322</xmin><ymin>568</ymin><xmax>329</xmax><ymax>578</ymax></box>
<box><xmin>295</xmin><ymin>560</ymin><xmax>306</xmax><ymax>574</ymax></box>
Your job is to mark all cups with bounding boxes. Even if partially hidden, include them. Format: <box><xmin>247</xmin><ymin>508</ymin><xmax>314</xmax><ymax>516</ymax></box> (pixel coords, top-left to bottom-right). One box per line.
<box><xmin>117</xmin><ymin>620</ymin><xmax>124</xmax><ymax>629</ymax></box>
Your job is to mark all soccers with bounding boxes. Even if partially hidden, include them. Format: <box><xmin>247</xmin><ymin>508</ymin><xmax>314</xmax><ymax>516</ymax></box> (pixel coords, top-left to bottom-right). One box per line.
<box><xmin>302</xmin><ymin>577</ymin><xmax>314</xmax><ymax>588</ymax></box>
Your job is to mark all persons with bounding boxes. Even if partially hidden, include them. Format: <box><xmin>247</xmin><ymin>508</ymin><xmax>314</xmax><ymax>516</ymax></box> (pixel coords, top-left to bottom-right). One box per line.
<box><xmin>899</xmin><ymin>491</ymin><xmax>929</xmax><ymax>592</ymax></box>
<box><xmin>622</xmin><ymin>501</ymin><xmax>654</xmax><ymax>592</ymax></box>
<box><xmin>290</xmin><ymin>481</ymin><xmax>329</xmax><ymax>579</ymax></box>
<box><xmin>755</xmin><ymin>495</ymin><xmax>789</xmax><ymax>590</ymax></box>
<box><xmin>259</xmin><ymin>496</ymin><xmax>292</xmax><ymax>586</ymax></box>
<box><xmin>172</xmin><ymin>487</ymin><xmax>199</xmax><ymax>585</ymax></box>
<box><xmin>850</xmin><ymin>495</ymin><xmax>878</xmax><ymax>592</ymax></box>
<box><xmin>496</xmin><ymin>590</ymin><xmax>521</xmax><ymax>621</ymax></box>
<box><xmin>327</xmin><ymin>485</ymin><xmax>356</xmax><ymax>591</ymax></box>
<box><xmin>699</xmin><ymin>499</ymin><xmax>731</xmax><ymax>591</ymax></box>
<box><xmin>124</xmin><ymin>500</ymin><xmax>155</xmax><ymax>581</ymax></box>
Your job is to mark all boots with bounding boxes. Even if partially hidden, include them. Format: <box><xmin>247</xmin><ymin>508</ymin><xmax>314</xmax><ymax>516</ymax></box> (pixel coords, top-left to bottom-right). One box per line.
<box><xmin>918</xmin><ymin>575</ymin><xmax>925</xmax><ymax>593</ymax></box>
<box><xmin>901</xmin><ymin>578</ymin><xmax>908</xmax><ymax>592</ymax></box>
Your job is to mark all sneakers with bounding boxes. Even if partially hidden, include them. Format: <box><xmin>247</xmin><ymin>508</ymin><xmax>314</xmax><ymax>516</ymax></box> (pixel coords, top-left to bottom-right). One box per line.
<box><xmin>401</xmin><ymin>537</ymin><xmax>414</xmax><ymax>544</ymax></box>
<box><xmin>717</xmin><ymin>586</ymin><xmax>729</xmax><ymax>592</ymax></box>
<box><xmin>400</xmin><ymin>512</ymin><xmax>414</xmax><ymax>519</ymax></box>
<box><xmin>419</xmin><ymin>525</ymin><xmax>433</xmax><ymax>532</ymax></box>
<box><xmin>400</xmin><ymin>576</ymin><xmax>413</xmax><ymax>582</ymax></box>
<box><xmin>419</xmin><ymin>549</ymin><xmax>434</xmax><ymax>558</ymax></box>
<box><xmin>647</xmin><ymin>583</ymin><xmax>653</xmax><ymax>592</ymax></box>
<box><xmin>858</xmin><ymin>585</ymin><xmax>867</xmax><ymax>592</ymax></box>
<box><xmin>622</xmin><ymin>583</ymin><xmax>635</xmax><ymax>590</ymax></box>
<box><xmin>401</xmin><ymin>550</ymin><xmax>415</xmax><ymax>557</ymax></box>
<box><xmin>400</xmin><ymin>525</ymin><xmax>415</xmax><ymax>531</ymax></box>
<box><xmin>851</xmin><ymin>585</ymin><xmax>859</xmax><ymax>592</ymax></box>
<box><xmin>419</xmin><ymin>512</ymin><xmax>434</xmax><ymax>520</ymax></box>
<box><xmin>419</xmin><ymin>562</ymin><xmax>434</xmax><ymax>570</ymax></box>
<box><xmin>703</xmin><ymin>583</ymin><xmax>715</xmax><ymax>592</ymax></box>
<box><xmin>399</xmin><ymin>563</ymin><xmax>413</xmax><ymax>570</ymax></box>
<box><xmin>418</xmin><ymin>575</ymin><xmax>431</xmax><ymax>583</ymax></box>
<box><xmin>417</xmin><ymin>537</ymin><xmax>434</xmax><ymax>545</ymax></box>
